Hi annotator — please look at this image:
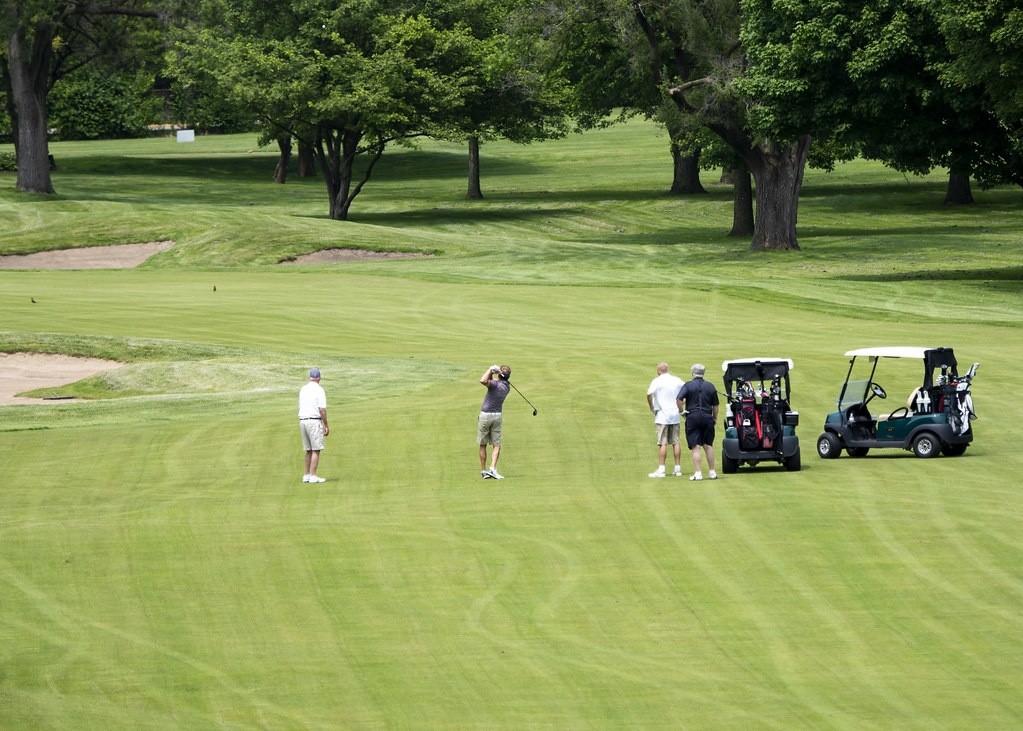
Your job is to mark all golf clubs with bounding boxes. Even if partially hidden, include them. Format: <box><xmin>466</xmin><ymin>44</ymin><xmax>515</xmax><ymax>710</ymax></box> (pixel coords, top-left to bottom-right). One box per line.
<box><xmin>498</xmin><ymin>371</ymin><xmax>538</xmax><ymax>417</ymax></box>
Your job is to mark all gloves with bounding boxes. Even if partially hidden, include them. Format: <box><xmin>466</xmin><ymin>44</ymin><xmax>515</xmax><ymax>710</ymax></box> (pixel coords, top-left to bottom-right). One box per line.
<box><xmin>680</xmin><ymin>410</ymin><xmax>689</xmax><ymax>420</ymax></box>
<box><xmin>490</xmin><ymin>365</ymin><xmax>500</xmax><ymax>372</ymax></box>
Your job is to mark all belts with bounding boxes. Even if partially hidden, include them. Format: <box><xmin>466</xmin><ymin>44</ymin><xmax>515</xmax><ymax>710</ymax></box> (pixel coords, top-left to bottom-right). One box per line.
<box><xmin>300</xmin><ymin>418</ymin><xmax>321</xmax><ymax>420</ymax></box>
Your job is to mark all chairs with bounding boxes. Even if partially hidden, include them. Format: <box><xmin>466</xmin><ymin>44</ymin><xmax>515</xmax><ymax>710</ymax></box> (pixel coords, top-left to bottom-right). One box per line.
<box><xmin>880</xmin><ymin>387</ymin><xmax>922</xmax><ymax>419</ymax></box>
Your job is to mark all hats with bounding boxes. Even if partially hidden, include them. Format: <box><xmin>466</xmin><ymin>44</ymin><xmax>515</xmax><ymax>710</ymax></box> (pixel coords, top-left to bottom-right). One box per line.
<box><xmin>691</xmin><ymin>364</ymin><xmax>705</xmax><ymax>374</ymax></box>
<box><xmin>500</xmin><ymin>366</ymin><xmax>511</xmax><ymax>374</ymax></box>
<box><xmin>310</xmin><ymin>368</ymin><xmax>320</xmax><ymax>378</ymax></box>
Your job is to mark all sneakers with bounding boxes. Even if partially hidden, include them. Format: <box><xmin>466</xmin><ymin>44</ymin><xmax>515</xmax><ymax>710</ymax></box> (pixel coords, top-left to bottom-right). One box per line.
<box><xmin>711</xmin><ymin>473</ymin><xmax>718</xmax><ymax>479</ymax></box>
<box><xmin>309</xmin><ymin>476</ymin><xmax>325</xmax><ymax>483</ymax></box>
<box><xmin>482</xmin><ymin>472</ymin><xmax>490</xmax><ymax>479</ymax></box>
<box><xmin>689</xmin><ymin>473</ymin><xmax>703</xmax><ymax>480</ymax></box>
<box><xmin>487</xmin><ymin>469</ymin><xmax>504</xmax><ymax>479</ymax></box>
<box><xmin>303</xmin><ymin>474</ymin><xmax>310</xmax><ymax>482</ymax></box>
<box><xmin>673</xmin><ymin>469</ymin><xmax>682</xmax><ymax>477</ymax></box>
<box><xmin>649</xmin><ymin>470</ymin><xmax>665</xmax><ymax>478</ymax></box>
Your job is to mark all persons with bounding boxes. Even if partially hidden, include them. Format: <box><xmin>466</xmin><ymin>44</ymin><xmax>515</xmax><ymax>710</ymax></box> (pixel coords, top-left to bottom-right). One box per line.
<box><xmin>476</xmin><ymin>365</ymin><xmax>511</xmax><ymax>479</ymax></box>
<box><xmin>298</xmin><ymin>368</ymin><xmax>329</xmax><ymax>484</ymax></box>
<box><xmin>676</xmin><ymin>364</ymin><xmax>719</xmax><ymax>480</ymax></box>
<box><xmin>646</xmin><ymin>362</ymin><xmax>686</xmax><ymax>478</ymax></box>
